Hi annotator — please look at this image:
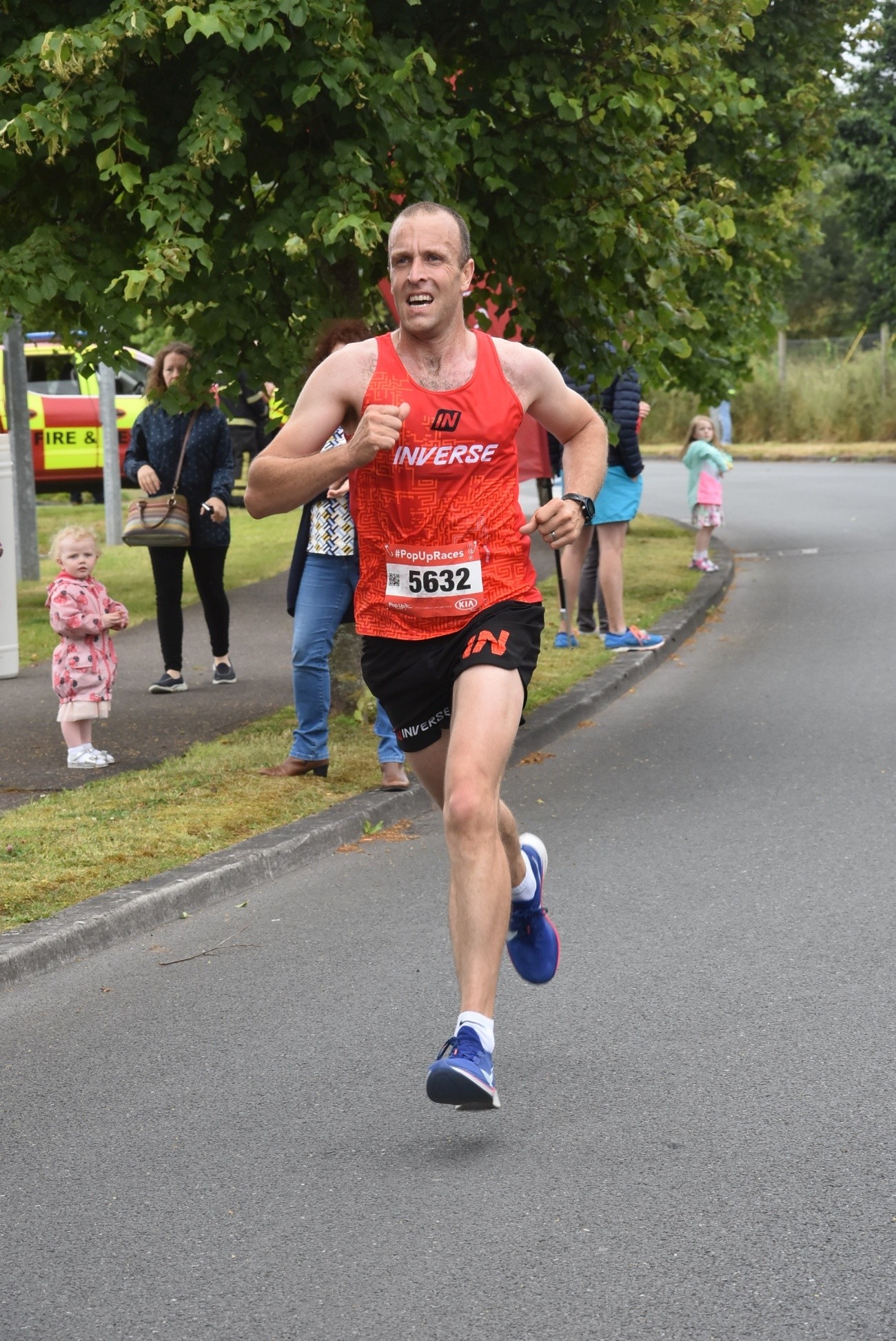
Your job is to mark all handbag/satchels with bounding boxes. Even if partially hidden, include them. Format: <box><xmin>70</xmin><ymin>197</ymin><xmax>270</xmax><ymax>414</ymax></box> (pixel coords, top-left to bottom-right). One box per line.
<box><xmin>121</xmin><ymin>494</ymin><xmax>191</xmax><ymax>548</ymax></box>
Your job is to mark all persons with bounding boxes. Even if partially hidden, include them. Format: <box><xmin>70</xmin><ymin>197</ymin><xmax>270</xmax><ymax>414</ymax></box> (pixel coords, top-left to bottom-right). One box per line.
<box><xmin>122</xmin><ymin>342</ymin><xmax>275</xmax><ymax>694</ymax></box>
<box><xmin>707</xmin><ymin>385</ymin><xmax>735</xmax><ymax>445</ymax></box>
<box><xmin>552</xmin><ymin>309</ymin><xmax>666</xmax><ymax>653</ymax></box>
<box><xmin>43</xmin><ymin>525</ymin><xmax>129</xmax><ymax>769</ymax></box>
<box><xmin>242</xmin><ymin>201</ymin><xmax>610</xmax><ymax>1112</ymax></box>
<box><xmin>679</xmin><ymin>415</ymin><xmax>734</xmax><ymax>572</ymax></box>
<box><xmin>255</xmin><ymin>318</ymin><xmax>411</xmax><ymax>792</ymax></box>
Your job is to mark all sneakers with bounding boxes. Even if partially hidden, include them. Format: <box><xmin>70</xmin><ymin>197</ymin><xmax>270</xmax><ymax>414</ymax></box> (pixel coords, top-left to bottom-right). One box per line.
<box><xmin>212</xmin><ymin>657</ymin><xmax>237</xmax><ymax>685</ymax></box>
<box><xmin>149</xmin><ymin>673</ymin><xmax>188</xmax><ymax>693</ymax></box>
<box><xmin>554</xmin><ymin>633</ymin><xmax>578</xmax><ymax>648</ymax></box>
<box><xmin>604</xmin><ymin>625</ymin><xmax>666</xmax><ymax>653</ymax></box>
<box><xmin>505</xmin><ymin>832</ymin><xmax>560</xmax><ymax>984</ymax></box>
<box><xmin>425</xmin><ymin>1025</ymin><xmax>501</xmax><ymax>1110</ymax></box>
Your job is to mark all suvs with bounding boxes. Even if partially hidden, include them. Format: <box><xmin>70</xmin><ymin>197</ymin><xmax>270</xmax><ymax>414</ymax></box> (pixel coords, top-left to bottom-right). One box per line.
<box><xmin>0</xmin><ymin>331</ymin><xmax>296</xmax><ymax>485</ymax></box>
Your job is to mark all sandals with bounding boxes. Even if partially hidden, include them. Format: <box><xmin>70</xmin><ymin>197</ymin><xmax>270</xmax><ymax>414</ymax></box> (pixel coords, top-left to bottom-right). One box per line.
<box><xmin>67</xmin><ymin>750</ymin><xmax>109</xmax><ymax>768</ymax></box>
<box><xmin>689</xmin><ymin>556</ymin><xmax>719</xmax><ymax>572</ymax></box>
<box><xmin>87</xmin><ymin>747</ymin><xmax>115</xmax><ymax>763</ymax></box>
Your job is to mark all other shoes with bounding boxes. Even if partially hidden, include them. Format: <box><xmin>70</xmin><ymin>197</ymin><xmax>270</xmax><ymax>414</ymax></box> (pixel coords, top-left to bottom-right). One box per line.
<box><xmin>599</xmin><ymin>634</ymin><xmax>606</xmax><ymax>641</ymax></box>
<box><xmin>578</xmin><ymin>627</ymin><xmax>601</xmax><ymax>636</ymax></box>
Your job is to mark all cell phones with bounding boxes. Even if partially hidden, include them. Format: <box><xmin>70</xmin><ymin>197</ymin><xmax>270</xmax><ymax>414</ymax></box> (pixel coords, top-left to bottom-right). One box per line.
<box><xmin>201</xmin><ymin>503</ymin><xmax>214</xmax><ymax>513</ymax></box>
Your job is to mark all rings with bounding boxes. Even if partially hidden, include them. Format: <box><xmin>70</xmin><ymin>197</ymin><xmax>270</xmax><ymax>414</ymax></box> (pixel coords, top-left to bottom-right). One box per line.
<box><xmin>551</xmin><ymin>531</ymin><xmax>557</xmax><ymax>540</ymax></box>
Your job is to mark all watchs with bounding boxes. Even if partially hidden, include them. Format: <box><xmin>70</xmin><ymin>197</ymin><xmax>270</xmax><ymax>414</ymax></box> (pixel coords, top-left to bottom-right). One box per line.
<box><xmin>561</xmin><ymin>493</ymin><xmax>596</xmax><ymax>525</ymax></box>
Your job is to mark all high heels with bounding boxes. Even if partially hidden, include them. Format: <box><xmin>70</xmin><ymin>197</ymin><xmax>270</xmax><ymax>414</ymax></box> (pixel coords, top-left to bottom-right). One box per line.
<box><xmin>380</xmin><ymin>762</ymin><xmax>410</xmax><ymax>791</ymax></box>
<box><xmin>258</xmin><ymin>755</ymin><xmax>330</xmax><ymax>778</ymax></box>
